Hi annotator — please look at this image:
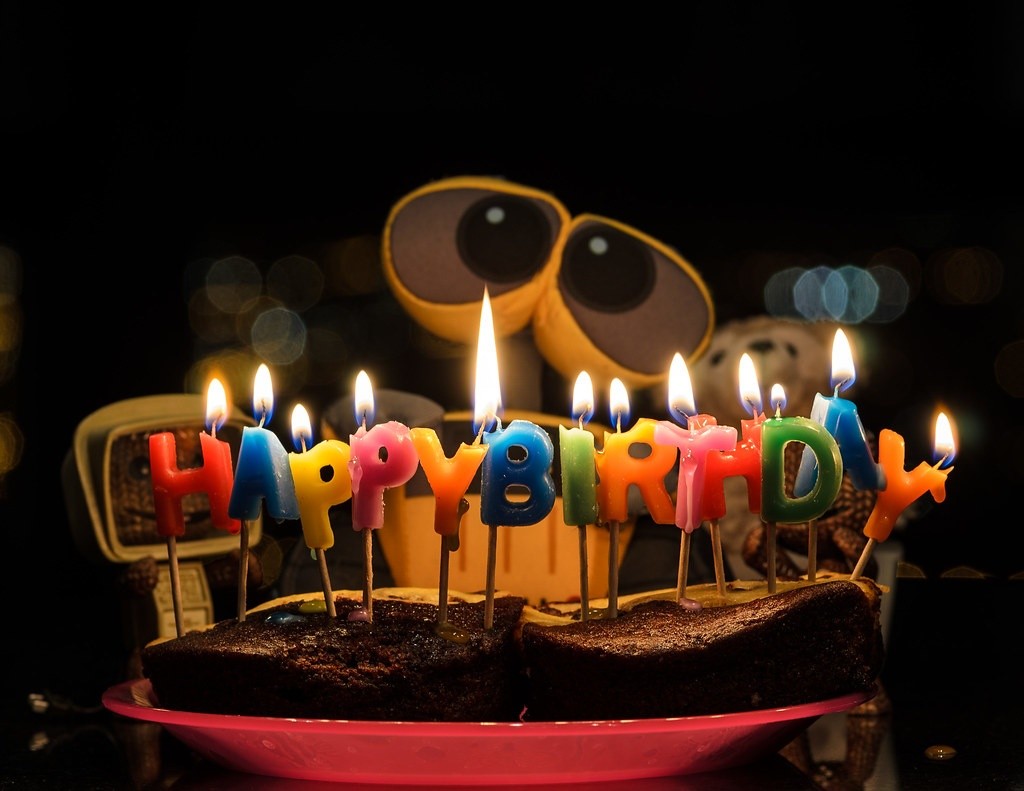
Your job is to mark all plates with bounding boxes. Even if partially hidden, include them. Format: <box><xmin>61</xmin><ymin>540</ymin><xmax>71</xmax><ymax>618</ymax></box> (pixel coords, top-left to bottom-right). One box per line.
<box><xmin>101</xmin><ymin>678</ymin><xmax>883</xmax><ymax>791</ymax></box>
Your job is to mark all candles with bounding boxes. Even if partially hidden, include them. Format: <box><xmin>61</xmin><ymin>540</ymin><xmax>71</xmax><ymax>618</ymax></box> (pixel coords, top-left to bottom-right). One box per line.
<box><xmin>348</xmin><ymin>368</ymin><xmax>419</xmax><ymax>624</ymax></box>
<box><xmin>761</xmin><ymin>382</ymin><xmax>843</xmax><ymax>595</ymax></box>
<box><xmin>469</xmin><ymin>285</ymin><xmax>555</xmax><ymax>627</ymax></box>
<box><xmin>558</xmin><ymin>370</ymin><xmax>597</xmax><ymax>621</ymax></box>
<box><xmin>598</xmin><ymin>377</ymin><xmax>678</xmax><ymax>620</ymax></box>
<box><xmin>147</xmin><ymin>365</ymin><xmax>241</xmax><ymax>641</ymax></box>
<box><xmin>280</xmin><ymin>396</ymin><xmax>350</xmax><ymax>618</ymax></box>
<box><xmin>225</xmin><ymin>363</ymin><xmax>299</xmax><ymax>621</ymax></box>
<box><xmin>653</xmin><ymin>351</ymin><xmax>738</xmax><ymax>601</ymax></box>
<box><xmin>793</xmin><ymin>325</ymin><xmax>887</xmax><ymax>582</ymax></box>
<box><xmin>408</xmin><ymin>424</ymin><xmax>490</xmax><ymax>621</ymax></box>
<box><xmin>687</xmin><ymin>352</ymin><xmax>761</xmax><ymax>596</ymax></box>
<box><xmin>849</xmin><ymin>404</ymin><xmax>960</xmax><ymax>585</ymax></box>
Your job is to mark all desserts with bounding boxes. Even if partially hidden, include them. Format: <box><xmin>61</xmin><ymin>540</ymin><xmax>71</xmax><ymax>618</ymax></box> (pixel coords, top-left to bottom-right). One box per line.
<box><xmin>141</xmin><ymin>585</ymin><xmax>534</xmax><ymax>723</ymax></box>
<box><xmin>521</xmin><ymin>568</ymin><xmax>886</xmax><ymax>721</ymax></box>
